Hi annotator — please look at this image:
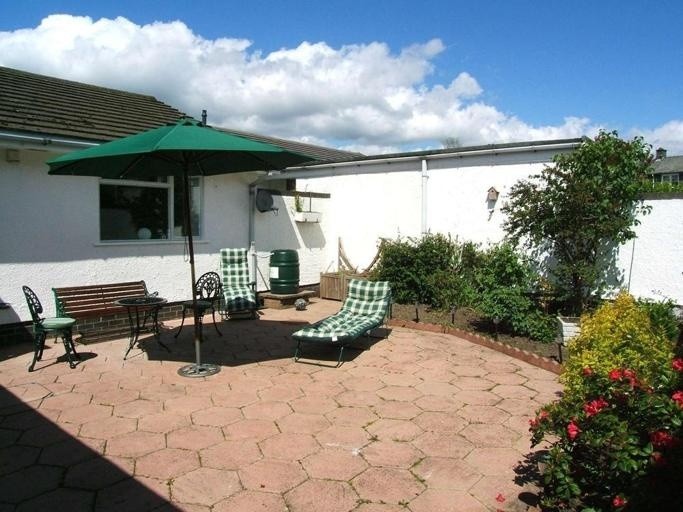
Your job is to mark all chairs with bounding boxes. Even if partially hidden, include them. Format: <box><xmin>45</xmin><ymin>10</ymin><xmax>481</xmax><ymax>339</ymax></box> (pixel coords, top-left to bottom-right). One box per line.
<box><xmin>293</xmin><ymin>279</ymin><xmax>392</xmax><ymax>367</ymax></box>
<box><xmin>175</xmin><ymin>272</ymin><xmax>222</xmax><ymax>339</ymax></box>
<box><xmin>22</xmin><ymin>285</ymin><xmax>80</xmax><ymax>371</ymax></box>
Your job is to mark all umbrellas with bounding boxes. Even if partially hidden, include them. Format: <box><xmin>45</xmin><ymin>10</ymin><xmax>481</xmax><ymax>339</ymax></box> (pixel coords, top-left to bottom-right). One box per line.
<box><xmin>46</xmin><ymin>116</ymin><xmax>316</xmax><ymax>378</ymax></box>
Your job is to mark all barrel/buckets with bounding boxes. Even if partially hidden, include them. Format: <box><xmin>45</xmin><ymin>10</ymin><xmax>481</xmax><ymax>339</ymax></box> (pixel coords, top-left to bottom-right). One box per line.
<box><xmin>269</xmin><ymin>248</ymin><xmax>301</xmax><ymax>295</ymax></box>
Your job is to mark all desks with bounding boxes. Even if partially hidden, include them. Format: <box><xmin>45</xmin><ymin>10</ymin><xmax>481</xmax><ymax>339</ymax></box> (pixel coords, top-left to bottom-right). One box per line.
<box><xmin>114</xmin><ymin>295</ymin><xmax>169</xmax><ymax>359</ymax></box>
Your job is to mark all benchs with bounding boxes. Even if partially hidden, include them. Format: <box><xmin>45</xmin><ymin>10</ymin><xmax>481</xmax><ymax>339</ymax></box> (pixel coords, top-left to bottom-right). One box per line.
<box><xmin>51</xmin><ymin>279</ymin><xmax>159</xmax><ymax>339</ymax></box>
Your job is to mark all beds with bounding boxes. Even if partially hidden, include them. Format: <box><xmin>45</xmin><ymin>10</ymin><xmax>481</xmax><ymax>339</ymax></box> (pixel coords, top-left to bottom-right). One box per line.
<box><xmin>219</xmin><ymin>247</ymin><xmax>259</xmax><ymax>321</ymax></box>
